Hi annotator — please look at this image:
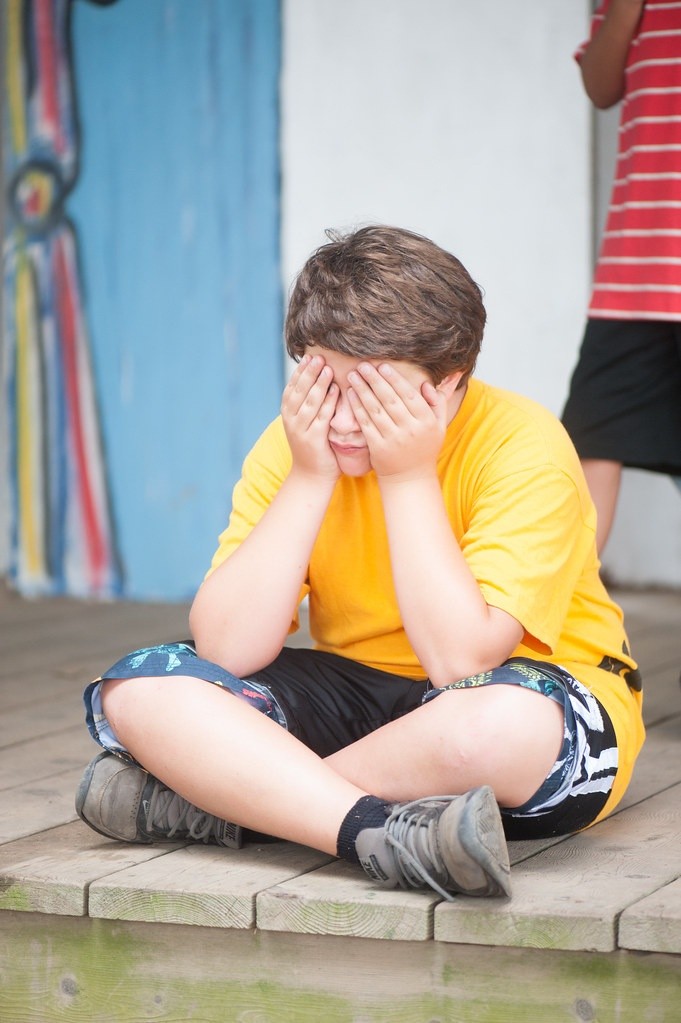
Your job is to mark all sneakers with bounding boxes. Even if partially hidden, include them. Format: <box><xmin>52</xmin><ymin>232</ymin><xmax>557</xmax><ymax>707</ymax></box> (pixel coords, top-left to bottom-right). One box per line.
<box><xmin>74</xmin><ymin>751</ymin><xmax>247</xmax><ymax>850</ymax></box>
<box><xmin>354</xmin><ymin>784</ymin><xmax>515</xmax><ymax>903</ymax></box>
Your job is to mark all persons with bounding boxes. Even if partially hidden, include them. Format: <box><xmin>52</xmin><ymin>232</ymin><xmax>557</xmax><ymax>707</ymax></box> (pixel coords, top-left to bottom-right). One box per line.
<box><xmin>560</xmin><ymin>1</ymin><xmax>681</xmax><ymax>559</ymax></box>
<box><xmin>73</xmin><ymin>224</ymin><xmax>646</xmax><ymax>901</ymax></box>
